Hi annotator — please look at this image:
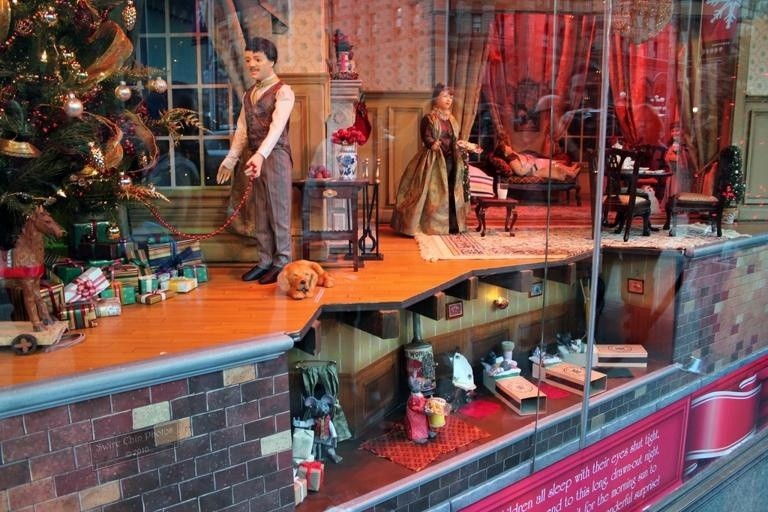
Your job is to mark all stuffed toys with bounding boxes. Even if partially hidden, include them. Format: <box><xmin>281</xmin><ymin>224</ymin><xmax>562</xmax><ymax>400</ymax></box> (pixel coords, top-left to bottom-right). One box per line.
<box><xmin>276</xmin><ymin>260</ymin><xmax>336</xmax><ymax>300</ymax></box>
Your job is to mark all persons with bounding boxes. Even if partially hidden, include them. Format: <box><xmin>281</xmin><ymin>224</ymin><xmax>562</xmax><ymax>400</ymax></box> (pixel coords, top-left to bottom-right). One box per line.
<box><xmin>390</xmin><ymin>82</ymin><xmax>485</xmax><ymax>237</ymax></box>
<box><xmin>493</xmin><ymin>141</ymin><xmax>582</xmax><ymax>181</ymax></box>
<box><xmin>215</xmin><ymin>36</ymin><xmax>297</xmax><ymax>284</ymax></box>
<box><xmin>660</xmin><ymin>120</ymin><xmax>700</xmax><ymax>212</ymax></box>
<box><xmin>566</xmin><ymin>64</ymin><xmax>603</xmax><ymax>112</ymax></box>
